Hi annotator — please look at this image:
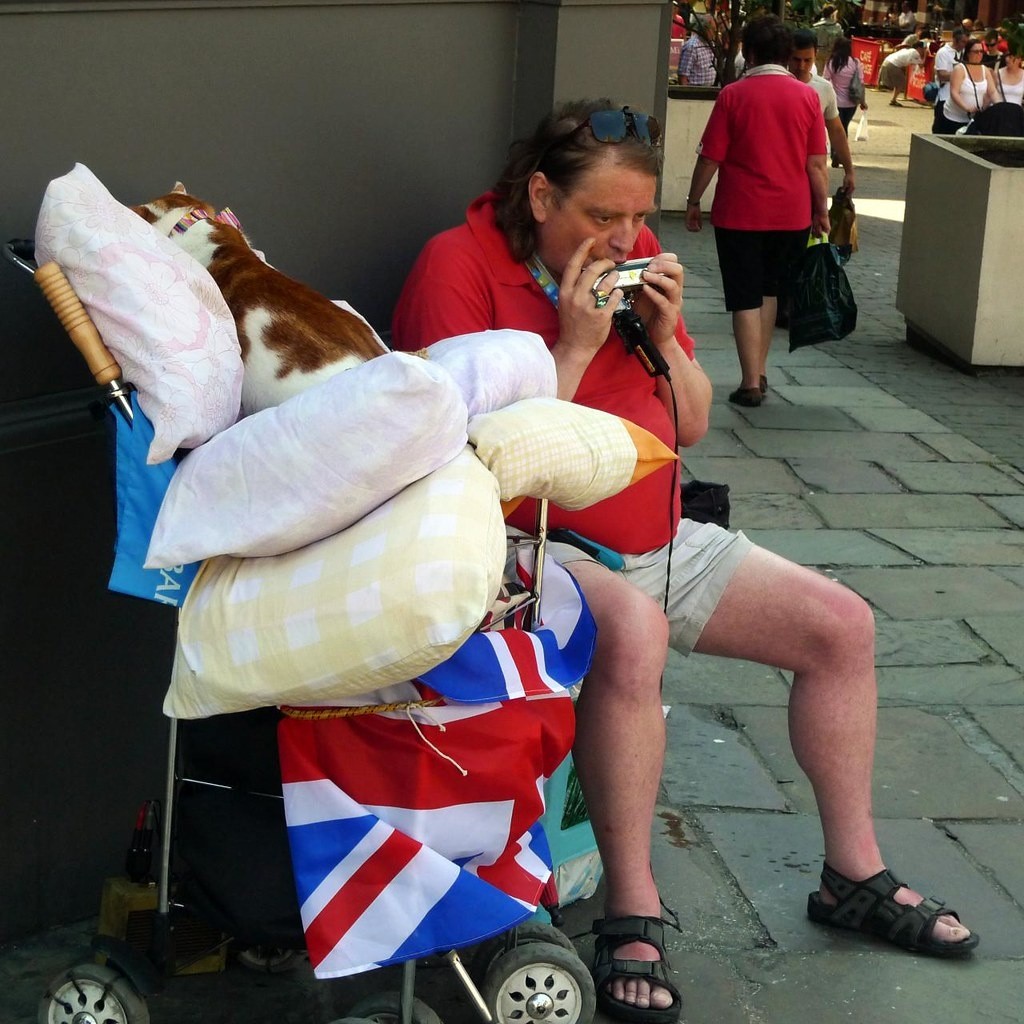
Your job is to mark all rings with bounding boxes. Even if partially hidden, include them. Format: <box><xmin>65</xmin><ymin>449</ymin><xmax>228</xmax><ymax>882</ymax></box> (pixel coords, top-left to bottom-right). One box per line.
<box><xmin>592</xmin><ymin>287</ymin><xmax>610</xmax><ymax>308</ymax></box>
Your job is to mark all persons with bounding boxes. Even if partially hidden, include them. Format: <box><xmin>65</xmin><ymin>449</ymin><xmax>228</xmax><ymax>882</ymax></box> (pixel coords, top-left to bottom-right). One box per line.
<box><xmin>678</xmin><ymin>0</ymin><xmax>818</xmax><ymax>77</ymax></box>
<box><xmin>670</xmin><ymin>0</ymin><xmax>686</xmax><ymax>40</ymax></box>
<box><xmin>932</xmin><ymin>39</ymin><xmax>1001</xmax><ymax>136</ymax></box>
<box><xmin>933</xmin><ymin>26</ymin><xmax>970</xmax><ymax>117</ymax></box>
<box><xmin>685</xmin><ymin>14</ymin><xmax>832</xmax><ymax>410</ymax></box>
<box><xmin>982</xmin><ymin>30</ymin><xmax>1007</xmax><ymax>69</ymax></box>
<box><xmin>853</xmin><ymin>0</ymin><xmax>1024</xmax><ymax>65</ymax></box>
<box><xmin>386</xmin><ymin>96</ymin><xmax>980</xmax><ymax>1024</ymax></box>
<box><xmin>878</xmin><ymin>41</ymin><xmax>929</xmax><ymax>108</ymax></box>
<box><xmin>720</xmin><ymin>30</ymin><xmax>746</xmax><ymax>88</ymax></box>
<box><xmin>984</xmin><ymin>44</ymin><xmax>1023</xmax><ymax>107</ymax></box>
<box><xmin>678</xmin><ymin>13</ymin><xmax>721</xmax><ymax>86</ymax></box>
<box><xmin>824</xmin><ymin>35</ymin><xmax>869</xmax><ymax>169</ymax></box>
<box><xmin>812</xmin><ymin>4</ymin><xmax>843</xmax><ymax>77</ymax></box>
<box><xmin>788</xmin><ymin>27</ymin><xmax>856</xmax><ymax>193</ymax></box>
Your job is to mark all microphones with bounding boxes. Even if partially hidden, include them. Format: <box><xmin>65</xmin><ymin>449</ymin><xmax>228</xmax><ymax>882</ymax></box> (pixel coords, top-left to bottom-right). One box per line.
<box><xmin>603</xmin><ymin>289</ymin><xmax>671</xmax><ymax>379</ymax></box>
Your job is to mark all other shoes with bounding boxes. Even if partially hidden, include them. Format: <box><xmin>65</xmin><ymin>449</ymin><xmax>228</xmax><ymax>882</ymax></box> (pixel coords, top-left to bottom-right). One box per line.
<box><xmin>728</xmin><ymin>387</ymin><xmax>762</xmax><ymax>406</ymax></box>
<box><xmin>890</xmin><ymin>99</ymin><xmax>903</xmax><ymax>107</ymax></box>
<box><xmin>759</xmin><ymin>375</ymin><xmax>768</xmax><ymax>392</ymax></box>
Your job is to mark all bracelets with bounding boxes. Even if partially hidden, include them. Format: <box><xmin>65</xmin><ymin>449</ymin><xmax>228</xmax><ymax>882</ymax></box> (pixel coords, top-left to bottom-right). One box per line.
<box><xmin>687</xmin><ymin>195</ymin><xmax>700</xmax><ymax>206</ymax></box>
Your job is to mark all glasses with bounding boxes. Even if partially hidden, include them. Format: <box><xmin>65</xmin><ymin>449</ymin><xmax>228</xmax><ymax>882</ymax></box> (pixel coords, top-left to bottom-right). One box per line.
<box><xmin>967</xmin><ymin>49</ymin><xmax>983</xmax><ymax>55</ymax></box>
<box><xmin>583</xmin><ymin>105</ymin><xmax>664</xmax><ymax>147</ymax></box>
<box><xmin>985</xmin><ymin>42</ymin><xmax>998</xmax><ymax>47</ymax></box>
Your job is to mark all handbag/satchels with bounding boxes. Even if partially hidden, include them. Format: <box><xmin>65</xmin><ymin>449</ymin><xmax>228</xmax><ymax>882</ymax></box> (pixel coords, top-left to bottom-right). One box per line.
<box><xmin>773</xmin><ymin>228</ymin><xmax>858</xmax><ymax>353</ymax></box>
<box><xmin>828</xmin><ymin>186</ymin><xmax>860</xmax><ymax>255</ymax></box>
<box><xmin>923</xmin><ymin>82</ymin><xmax>939</xmax><ymax>102</ymax></box>
<box><xmin>848</xmin><ymin>58</ymin><xmax>867</xmax><ymax>104</ymax></box>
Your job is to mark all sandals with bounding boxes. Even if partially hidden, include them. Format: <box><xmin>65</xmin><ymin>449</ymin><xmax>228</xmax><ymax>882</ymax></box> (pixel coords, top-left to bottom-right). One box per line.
<box><xmin>590</xmin><ymin>892</ymin><xmax>683</xmax><ymax>1022</ymax></box>
<box><xmin>806</xmin><ymin>859</ymin><xmax>983</xmax><ymax>958</ymax></box>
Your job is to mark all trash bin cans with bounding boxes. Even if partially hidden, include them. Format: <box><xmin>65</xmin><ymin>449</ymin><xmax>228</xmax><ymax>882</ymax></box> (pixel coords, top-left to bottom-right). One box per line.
<box><xmin>893</xmin><ymin>131</ymin><xmax>1024</xmax><ymax>378</ymax></box>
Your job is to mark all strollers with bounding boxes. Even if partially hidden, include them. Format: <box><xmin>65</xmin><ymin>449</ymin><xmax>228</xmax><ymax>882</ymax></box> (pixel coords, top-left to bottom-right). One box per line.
<box><xmin>1</xmin><ymin>237</ymin><xmax>600</xmax><ymax>1024</ymax></box>
<box><xmin>968</xmin><ymin>100</ymin><xmax>1024</xmax><ymax>138</ymax></box>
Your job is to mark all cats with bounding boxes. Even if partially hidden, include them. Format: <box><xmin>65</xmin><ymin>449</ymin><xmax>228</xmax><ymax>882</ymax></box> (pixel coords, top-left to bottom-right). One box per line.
<box><xmin>127</xmin><ymin>180</ymin><xmax>390</xmax><ymax>420</ymax></box>
